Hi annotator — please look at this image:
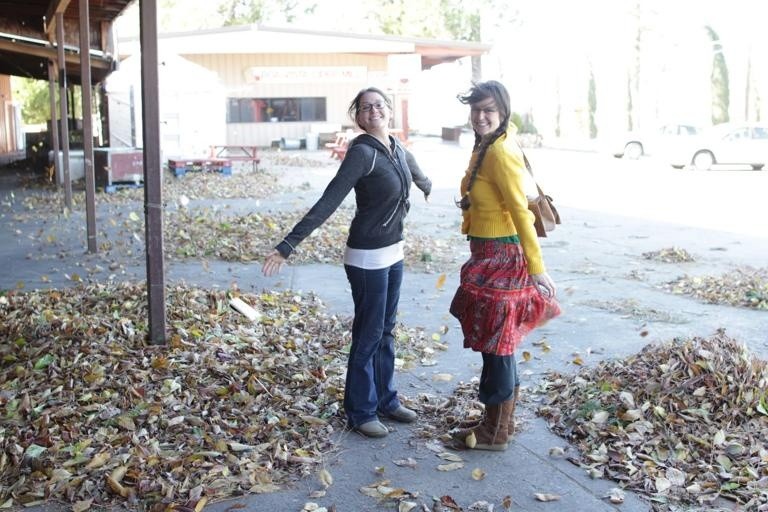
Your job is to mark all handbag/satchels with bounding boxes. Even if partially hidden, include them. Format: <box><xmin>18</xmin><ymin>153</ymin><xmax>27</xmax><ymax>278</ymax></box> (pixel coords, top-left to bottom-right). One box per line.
<box><xmin>528</xmin><ymin>195</ymin><xmax>562</xmax><ymax>238</ymax></box>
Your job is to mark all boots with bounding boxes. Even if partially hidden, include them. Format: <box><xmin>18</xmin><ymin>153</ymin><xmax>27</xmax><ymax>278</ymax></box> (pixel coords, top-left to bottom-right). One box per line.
<box><xmin>452</xmin><ymin>384</ymin><xmax>520</xmax><ymax>450</ymax></box>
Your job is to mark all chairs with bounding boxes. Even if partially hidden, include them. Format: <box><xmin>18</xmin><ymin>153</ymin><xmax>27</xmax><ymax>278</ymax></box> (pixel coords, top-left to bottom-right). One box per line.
<box><xmin>324</xmin><ymin>132</ymin><xmax>351</xmax><ymax>161</ymax></box>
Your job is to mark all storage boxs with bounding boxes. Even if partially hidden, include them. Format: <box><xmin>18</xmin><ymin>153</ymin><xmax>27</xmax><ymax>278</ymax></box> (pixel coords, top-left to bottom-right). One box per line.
<box><xmin>93</xmin><ymin>146</ymin><xmax>145</xmax><ymax>186</ymax></box>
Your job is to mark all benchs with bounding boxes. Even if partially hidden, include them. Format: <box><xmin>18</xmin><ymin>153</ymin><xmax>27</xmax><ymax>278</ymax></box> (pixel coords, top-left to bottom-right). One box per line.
<box><xmin>209</xmin><ymin>158</ymin><xmax>259</xmax><ymax>174</ymax></box>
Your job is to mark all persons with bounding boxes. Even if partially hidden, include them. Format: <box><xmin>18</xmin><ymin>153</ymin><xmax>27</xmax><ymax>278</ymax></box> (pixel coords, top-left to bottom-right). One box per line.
<box><xmin>453</xmin><ymin>81</ymin><xmax>562</xmax><ymax>450</ymax></box>
<box><xmin>261</xmin><ymin>88</ymin><xmax>432</xmax><ymax>437</ymax></box>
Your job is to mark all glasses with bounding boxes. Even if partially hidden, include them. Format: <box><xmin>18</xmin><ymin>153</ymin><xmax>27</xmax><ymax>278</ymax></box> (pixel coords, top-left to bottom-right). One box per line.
<box><xmin>358</xmin><ymin>101</ymin><xmax>385</xmax><ymax>112</ymax></box>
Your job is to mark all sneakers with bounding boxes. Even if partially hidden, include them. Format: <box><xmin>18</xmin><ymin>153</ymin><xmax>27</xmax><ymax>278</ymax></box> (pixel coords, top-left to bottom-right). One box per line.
<box><xmin>389</xmin><ymin>405</ymin><xmax>416</xmax><ymax>422</ymax></box>
<box><xmin>358</xmin><ymin>420</ymin><xmax>389</xmax><ymax>437</ymax></box>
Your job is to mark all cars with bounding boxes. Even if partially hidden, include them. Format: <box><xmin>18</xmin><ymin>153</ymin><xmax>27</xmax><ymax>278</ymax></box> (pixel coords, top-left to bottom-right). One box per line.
<box><xmin>658</xmin><ymin>121</ymin><xmax>767</xmax><ymax>173</ymax></box>
<box><xmin>610</xmin><ymin>118</ymin><xmax>708</xmax><ymax>173</ymax></box>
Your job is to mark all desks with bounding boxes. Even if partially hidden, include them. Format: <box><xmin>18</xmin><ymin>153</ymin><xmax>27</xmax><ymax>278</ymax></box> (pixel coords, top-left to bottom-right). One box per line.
<box><xmin>208</xmin><ymin>143</ymin><xmax>261</xmax><ymax>173</ymax></box>
